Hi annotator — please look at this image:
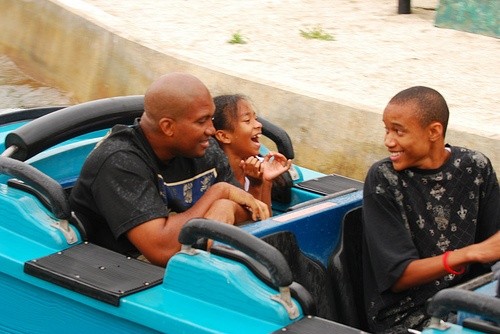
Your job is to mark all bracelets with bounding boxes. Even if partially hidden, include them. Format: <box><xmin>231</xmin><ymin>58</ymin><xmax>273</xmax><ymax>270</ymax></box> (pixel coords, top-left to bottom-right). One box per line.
<box><xmin>442</xmin><ymin>251</ymin><xmax>465</xmax><ymax>275</ymax></box>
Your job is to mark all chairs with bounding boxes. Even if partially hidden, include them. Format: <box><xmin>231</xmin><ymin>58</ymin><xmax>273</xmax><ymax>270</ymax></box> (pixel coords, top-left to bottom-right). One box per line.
<box><xmin>328</xmin><ymin>206</ymin><xmax>363</xmax><ymax>328</ymax></box>
<box><xmin>260</xmin><ymin>232</ymin><xmax>340</xmax><ymax>322</ymax></box>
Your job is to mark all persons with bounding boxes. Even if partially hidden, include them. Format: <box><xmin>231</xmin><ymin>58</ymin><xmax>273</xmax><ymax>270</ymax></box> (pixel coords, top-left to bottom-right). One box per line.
<box><xmin>210</xmin><ymin>94</ymin><xmax>291</xmax><ymax>217</ymax></box>
<box><xmin>362</xmin><ymin>86</ymin><xmax>500</xmax><ymax>334</ymax></box>
<box><xmin>69</xmin><ymin>72</ymin><xmax>269</xmax><ymax>268</ymax></box>
